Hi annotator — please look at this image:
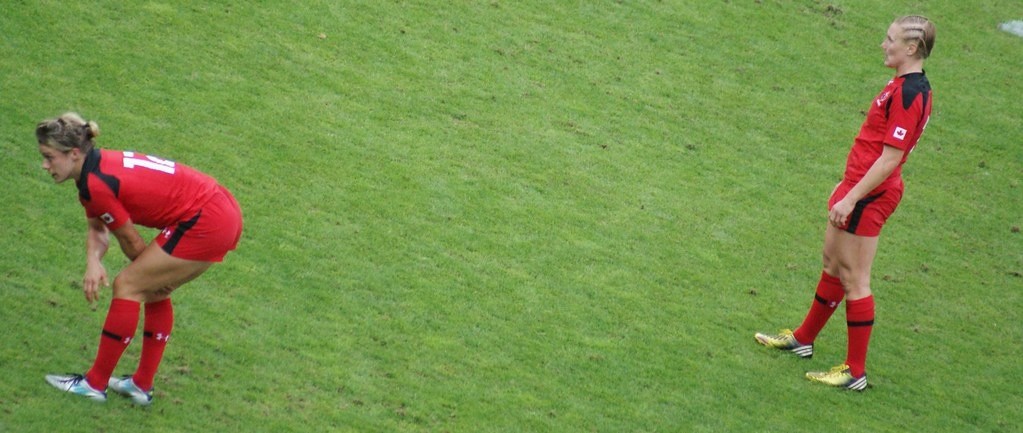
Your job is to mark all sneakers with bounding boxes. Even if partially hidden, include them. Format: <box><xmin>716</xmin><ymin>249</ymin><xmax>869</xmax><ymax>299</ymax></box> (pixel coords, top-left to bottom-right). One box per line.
<box><xmin>45</xmin><ymin>373</ymin><xmax>108</xmax><ymax>402</ymax></box>
<box><xmin>108</xmin><ymin>375</ymin><xmax>154</xmax><ymax>406</ymax></box>
<box><xmin>805</xmin><ymin>364</ymin><xmax>868</xmax><ymax>392</ymax></box>
<box><xmin>754</xmin><ymin>329</ymin><xmax>814</xmax><ymax>359</ymax></box>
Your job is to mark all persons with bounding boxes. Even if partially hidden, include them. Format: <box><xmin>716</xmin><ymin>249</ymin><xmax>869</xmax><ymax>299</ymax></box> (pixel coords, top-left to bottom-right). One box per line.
<box><xmin>754</xmin><ymin>14</ymin><xmax>936</xmax><ymax>395</ymax></box>
<box><xmin>36</xmin><ymin>112</ymin><xmax>244</xmax><ymax>405</ymax></box>
